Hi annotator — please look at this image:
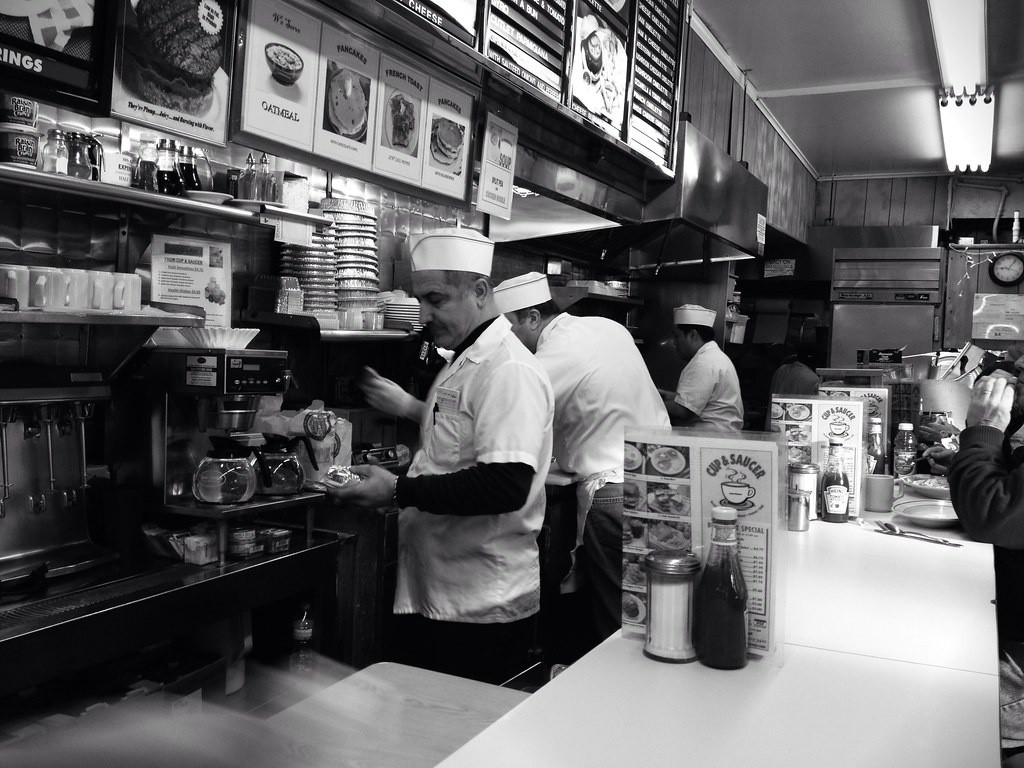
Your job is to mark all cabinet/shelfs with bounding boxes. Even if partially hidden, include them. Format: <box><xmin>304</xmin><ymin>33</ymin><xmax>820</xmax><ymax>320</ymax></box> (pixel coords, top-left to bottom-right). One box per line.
<box><xmin>0</xmin><ymin>162</ymin><xmax>260</xmax><ymax>328</ymax></box>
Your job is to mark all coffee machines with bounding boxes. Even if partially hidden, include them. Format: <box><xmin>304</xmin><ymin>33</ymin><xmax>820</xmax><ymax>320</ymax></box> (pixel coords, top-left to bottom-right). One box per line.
<box><xmin>166</xmin><ymin>350</ymin><xmax>293</xmax><ymax>432</ymax></box>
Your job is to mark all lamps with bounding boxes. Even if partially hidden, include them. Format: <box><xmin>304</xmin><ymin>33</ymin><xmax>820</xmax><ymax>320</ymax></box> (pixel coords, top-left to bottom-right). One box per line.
<box><xmin>926</xmin><ymin>0</ymin><xmax>999</xmax><ymax>174</ymax></box>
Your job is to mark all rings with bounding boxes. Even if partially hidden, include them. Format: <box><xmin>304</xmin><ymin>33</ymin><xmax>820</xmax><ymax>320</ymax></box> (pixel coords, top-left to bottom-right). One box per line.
<box><xmin>982</xmin><ymin>390</ymin><xmax>991</xmax><ymax>394</ymax></box>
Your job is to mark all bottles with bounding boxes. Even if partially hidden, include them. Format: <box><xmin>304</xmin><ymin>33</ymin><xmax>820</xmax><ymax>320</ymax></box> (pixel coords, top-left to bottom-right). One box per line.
<box><xmin>642</xmin><ymin>506</ymin><xmax>747</xmax><ymax>670</ymax></box>
<box><xmin>131</xmin><ymin>132</ymin><xmax>214</xmax><ymax>197</ymax></box>
<box><xmin>893</xmin><ymin>423</ymin><xmax>917</xmax><ymax>479</ymax></box>
<box><xmin>237</xmin><ymin>153</ymin><xmax>278</xmax><ymax>202</ymax></box>
<box><xmin>866</xmin><ymin>418</ymin><xmax>885</xmax><ymax>474</ymax></box>
<box><xmin>787</xmin><ymin>437</ymin><xmax>849</xmax><ymax>531</ymax></box>
<box><xmin>289</xmin><ymin>622</ymin><xmax>314</xmax><ymax>679</ymax></box>
<box><xmin>42</xmin><ymin>129</ymin><xmax>69</xmax><ymax>176</ymax></box>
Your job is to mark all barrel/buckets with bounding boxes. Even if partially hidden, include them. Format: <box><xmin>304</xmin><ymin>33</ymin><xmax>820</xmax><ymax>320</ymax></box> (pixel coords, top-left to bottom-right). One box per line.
<box><xmin>0</xmin><ymin>122</ymin><xmax>44</xmax><ymax>171</ymax></box>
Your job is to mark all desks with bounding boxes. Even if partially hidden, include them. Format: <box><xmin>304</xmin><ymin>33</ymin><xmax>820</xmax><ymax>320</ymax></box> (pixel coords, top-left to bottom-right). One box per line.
<box><xmin>434</xmin><ymin>477</ymin><xmax>1002</xmax><ymax>768</ymax></box>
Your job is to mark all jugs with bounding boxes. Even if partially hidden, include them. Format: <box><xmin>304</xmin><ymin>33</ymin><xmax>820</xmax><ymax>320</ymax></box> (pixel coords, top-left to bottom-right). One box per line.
<box><xmin>66</xmin><ymin>132</ymin><xmax>106</xmax><ymax>183</ymax></box>
<box><xmin>191</xmin><ymin>435</ymin><xmax>272</xmax><ymax>504</ymax></box>
<box><xmin>254</xmin><ymin>432</ymin><xmax>320</xmax><ymax>496</ymax></box>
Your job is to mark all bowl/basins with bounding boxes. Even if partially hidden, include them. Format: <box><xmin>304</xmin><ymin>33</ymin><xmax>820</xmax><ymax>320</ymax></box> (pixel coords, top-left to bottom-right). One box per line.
<box><xmin>265</xmin><ymin>42</ymin><xmax>304</xmax><ymax>86</ymax></box>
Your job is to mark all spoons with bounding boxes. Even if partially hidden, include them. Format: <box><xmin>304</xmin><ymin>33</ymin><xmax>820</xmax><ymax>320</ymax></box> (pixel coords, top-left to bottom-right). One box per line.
<box><xmin>884</xmin><ymin>523</ymin><xmax>963</xmax><ymax>547</ymax></box>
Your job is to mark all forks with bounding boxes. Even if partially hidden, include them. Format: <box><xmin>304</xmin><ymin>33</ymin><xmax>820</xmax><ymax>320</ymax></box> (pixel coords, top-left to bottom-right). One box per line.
<box><xmin>875</xmin><ymin>520</ymin><xmax>964</xmax><ymax>547</ymax></box>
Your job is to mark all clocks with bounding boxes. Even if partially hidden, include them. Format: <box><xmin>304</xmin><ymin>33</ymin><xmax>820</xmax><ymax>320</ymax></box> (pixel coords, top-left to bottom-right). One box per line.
<box><xmin>988</xmin><ymin>252</ymin><xmax>1024</xmax><ymax>288</ymax></box>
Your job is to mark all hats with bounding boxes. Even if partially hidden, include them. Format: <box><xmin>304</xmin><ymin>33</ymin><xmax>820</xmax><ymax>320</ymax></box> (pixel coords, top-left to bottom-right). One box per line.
<box><xmin>493</xmin><ymin>271</ymin><xmax>552</xmax><ymax>313</ymax></box>
<box><xmin>673</xmin><ymin>304</ymin><xmax>718</xmax><ymax>328</ymax></box>
<box><xmin>408</xmin><ymin>227</ymin><xmax>495</xmax><ymax>277</ymax></box>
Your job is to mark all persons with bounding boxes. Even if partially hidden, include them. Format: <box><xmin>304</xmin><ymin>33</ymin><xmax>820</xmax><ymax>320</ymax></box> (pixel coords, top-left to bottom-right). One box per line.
<box><xmin>922</xmin><ymin>344</ymin><xmax>1024</xmax><ymax>475</ymax></box>
<box><xmin>326</xmin><ymin>227</ymin><xmax>555</xmax><ymax>685</ymax></box>
<box><xmin>658</xmin><ymin>303</ymin><xmax>744</xmax><ymax>432</ymax></box>
<box><xmin>764</xmin><ymin>342</ymin><xmax>819</xmax><ymax>431</ymax></box>
<box><xmin>945</xmin><ymin>352</ymin><xmax>1024</xmax><ymax>749</ymax></box>
<box><xmin>361</xmin><ymin>272</ymin><xmax>673</xmax><ymax>641</ymax></box>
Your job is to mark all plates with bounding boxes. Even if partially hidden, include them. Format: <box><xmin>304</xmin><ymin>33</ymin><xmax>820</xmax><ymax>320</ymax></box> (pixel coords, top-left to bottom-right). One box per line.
<box><xmin>384</xmin><ymin>297</ymin><xmax>423</xmax><ymax>331</ymax></box>
<box><xmin>319</xmin><ymin>198</ymin><xmax>380</xmax><ymax>312</ymax></box>
<box><xmin>275</xmin><ymin>213</ymin><xmax>338</xmax><ymax>314</ymax></box>
<box><xmin>184</xmin><ymin>190</ymin><xmax>234</xmax><ymax>206</ymax></box>
<box><xmin>771</xmin><ymin>404</ymin><xmax>810</xmax><ymax>441</ymax></box>
<box><xmin>230</xmin><ymin>198</ymin><xmax>286</xmax><ymax>213</ymax></box>
<box><xmin>892</xmin><ymin>499</ymin><xmax>959</xmax><ymax>528</ymax></box>
<box><xmin>622</xmin><ymin>592</ymin><xmax>647</xmax><ymax>624</ymax></box>
<box><xmin>902</xmin><ymin>475</ymin><xmax>951</xmax><ymax>500</ymax></box>
<box><xmin>325</xmin><ymin>70</ymin><xmax>420</xmax><ymax>155</ymax></box>
<box><xmin>624</xmin><ymin>443</ymin><xmax>686</xmax><ymax>514</ymax></box>
<box><xmin>178</xmin><ymin>328</ymin><xmax>260</xmax><ymax>350</ymax></box>
<box><xmin>112</xmin><ymin>66</ymin><xmax>229</xmax><ymax>143</ymax></box>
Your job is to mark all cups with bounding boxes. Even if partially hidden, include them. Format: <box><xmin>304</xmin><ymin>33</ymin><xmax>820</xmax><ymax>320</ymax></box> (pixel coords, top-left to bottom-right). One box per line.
<box><xmin>0</xmin><ymin>263</ymin><xmax>141</xmax><ymax>311</ymax></box>
<box><xmin>865</xmin><ymin>474</ymin><xmax>904</xmax><ymax>512</ymax></box>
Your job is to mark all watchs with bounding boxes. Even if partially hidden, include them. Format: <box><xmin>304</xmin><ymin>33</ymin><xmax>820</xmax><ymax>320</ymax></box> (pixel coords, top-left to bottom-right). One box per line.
<box><xmin>389</xmin><ymin>479</ymin><xmax>399</xmax><ymax>508</ymax></box>
